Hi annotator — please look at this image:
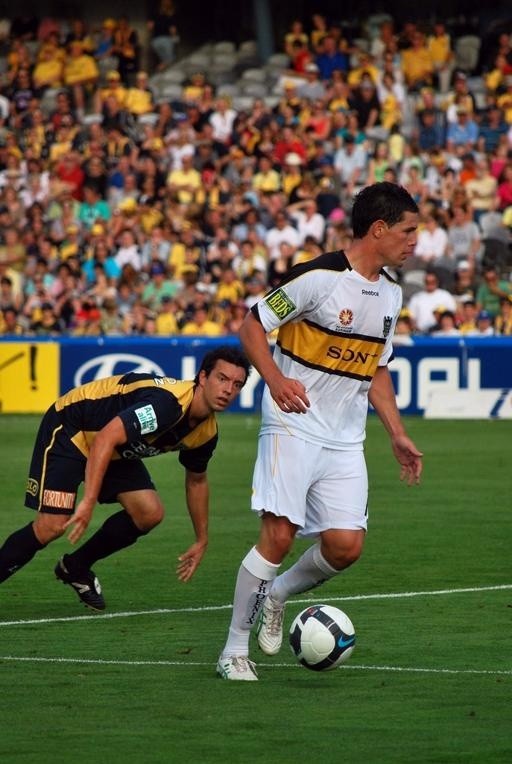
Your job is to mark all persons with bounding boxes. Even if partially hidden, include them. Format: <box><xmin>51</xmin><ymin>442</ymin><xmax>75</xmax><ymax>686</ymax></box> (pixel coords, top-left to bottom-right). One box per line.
<box><xmin>0</xmin><ymin>345</ymin><xmax>253</xmax><ymax>612</ymax></box>
<box><xmin>214</xmin><ymin>179</ymin><xmax>424</xmax><ymax>682</ymax></box>
<box><xmin>0</xmin><ymin>1</ymin><xmax>512</xmax><ymax>341</ymax></box>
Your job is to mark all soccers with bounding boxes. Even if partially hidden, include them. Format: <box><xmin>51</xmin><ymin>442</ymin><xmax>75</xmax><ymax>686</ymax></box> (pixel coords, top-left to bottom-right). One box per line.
<box><xmin>289</xmin><ymin>604</ymin><xmax>357</xmax><ymax>670</ymax></box>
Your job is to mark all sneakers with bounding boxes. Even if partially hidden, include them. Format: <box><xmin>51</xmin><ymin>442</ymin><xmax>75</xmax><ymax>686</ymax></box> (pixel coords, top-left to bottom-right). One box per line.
<box><xmin>256</xmin><ymin>594</ymin><xmax>285</xmax><ymax>654</ymax></box>
<box><xmin>217</xmin><ymin>650</ymin><xmax>260</xmax><ymax>683</ymax></box>
<box><xmin>55</xmin><ymin>552</ymin><xmax>106</xmax><ymax>611</ymax></box>
